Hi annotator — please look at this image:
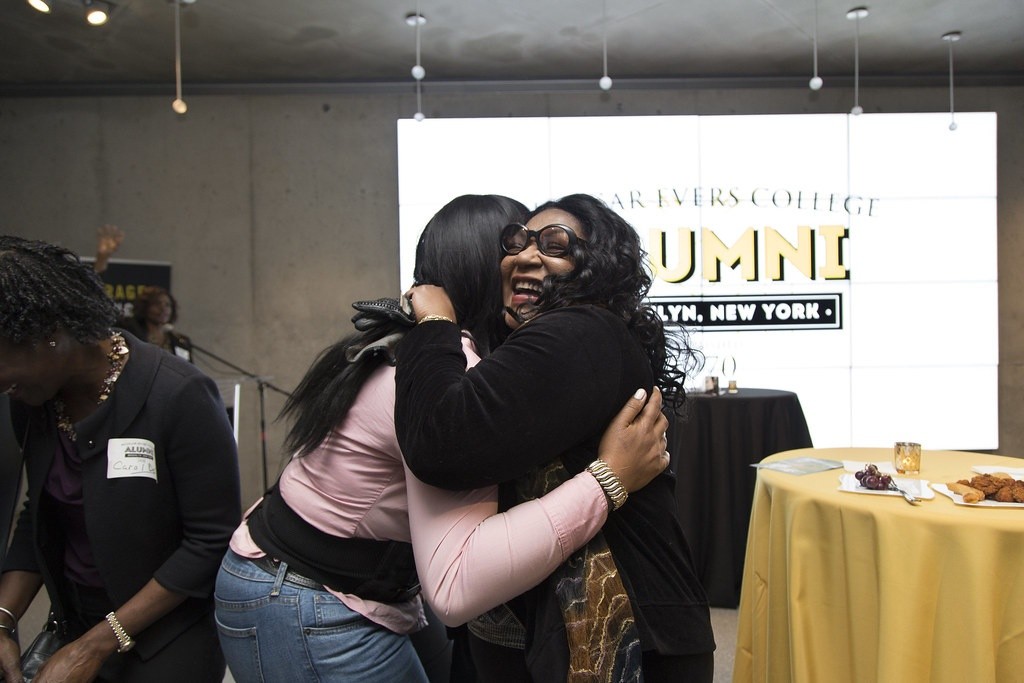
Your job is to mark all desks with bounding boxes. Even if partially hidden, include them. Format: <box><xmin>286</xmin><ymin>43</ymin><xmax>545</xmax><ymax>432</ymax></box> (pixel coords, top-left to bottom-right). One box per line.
<box><xmin>734</xmin><ymin>447</ymin><xmax>1024</xmax><ymax>683</ymax></box>
<box><xmin>669</xmin><ymin>388</ymin><xmax>813</xmax><ymax>610</ymax></box>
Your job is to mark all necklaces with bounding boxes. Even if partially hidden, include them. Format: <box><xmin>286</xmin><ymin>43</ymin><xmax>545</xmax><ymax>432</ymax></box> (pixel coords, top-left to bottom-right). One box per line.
<box><xmin>51</xmin><ymin>330</ymin><xmax>129</xmax><ymax>443</ymax></box>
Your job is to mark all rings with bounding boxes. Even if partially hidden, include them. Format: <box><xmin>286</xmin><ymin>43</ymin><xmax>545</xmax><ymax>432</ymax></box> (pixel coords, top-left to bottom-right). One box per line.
<box><xmin>662</xmin><ymin>456</ymin><xmax>669</xmax><ymax>464</ymax></box>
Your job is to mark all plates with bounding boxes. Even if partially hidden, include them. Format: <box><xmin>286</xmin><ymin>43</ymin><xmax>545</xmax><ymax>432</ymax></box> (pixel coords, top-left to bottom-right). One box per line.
<box><xmin>931</xmin><ymin>483</ymin><xmax>1024</xmax><ymax>508</ymax></box>
<box><xmin>837</xmin><ymin>473</ymin><xmax>935</xmax><ymax>500</ymax></box>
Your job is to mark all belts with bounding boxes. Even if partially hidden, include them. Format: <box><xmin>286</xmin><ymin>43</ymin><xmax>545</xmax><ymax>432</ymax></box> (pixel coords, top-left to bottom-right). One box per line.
<box><xmin>252</xmin><ymin>553</ymin><xmax>326</xmax><ymax>593</ymax></box>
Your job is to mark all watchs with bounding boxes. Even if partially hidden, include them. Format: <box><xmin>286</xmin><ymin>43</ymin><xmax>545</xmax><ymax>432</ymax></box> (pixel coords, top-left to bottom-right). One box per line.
<box><xmin>105</xmin><ymin>612</ymin><xmax>135</xmax><ymax>653</ymax></box>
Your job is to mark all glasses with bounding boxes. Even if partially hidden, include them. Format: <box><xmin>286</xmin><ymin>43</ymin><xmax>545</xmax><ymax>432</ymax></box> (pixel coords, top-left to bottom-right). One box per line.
<box><xmin>501</xmin><ymin>223</ymin><xmax>589</xmax><ymax>258</ymax></box>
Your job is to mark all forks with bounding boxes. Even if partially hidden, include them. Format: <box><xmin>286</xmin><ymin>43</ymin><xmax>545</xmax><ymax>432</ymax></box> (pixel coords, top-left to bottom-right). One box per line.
<box><xmin>886</xmin><ymin>475</ymin><xmax>922</xmax><ymax>506</ymax></box>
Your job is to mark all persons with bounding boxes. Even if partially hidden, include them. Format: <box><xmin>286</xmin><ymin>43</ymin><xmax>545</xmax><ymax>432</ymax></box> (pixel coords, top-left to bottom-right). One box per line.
<box><xmin>0</xmin><ymin>236</ymin><xmax>242</xmax><ymax>683</ymax></box>
<box><xmin>95</xmin><ymin>225</ymin><xmax>193</xmax><ymax>366</ymax></box>
<box><xmin>214</xmin><ymin>194</ymin><xmax>670</xmax><ymax>683</ymax></box>
<box><xmin>395</xmin><ymin>193</ymin><xmax>716</xmax><ymax>683</ymax></box>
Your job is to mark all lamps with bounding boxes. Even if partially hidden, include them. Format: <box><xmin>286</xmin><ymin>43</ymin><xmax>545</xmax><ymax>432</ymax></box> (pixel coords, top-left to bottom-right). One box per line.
<box><xmin>943</xmin><ymin>31</ymin><xmax>960</xmax><ymax>131</ymax></box>
<box><xmin>807</xmin><ymin>1</ymin><xmax>822</xmax><ymax>92</ymax></box>
<box><xmin>844</xmin><ymin>7</ymin><xmax>869</xmax><ymax>117</ymax></box>
<box><xmin>170</xmin><ymin>0</ymin><xmax>188</xmax><ymax>114</ymax></box>
<box><xmin>411</xmin><ymin>0</ymin><xmax>426</xmax><ymax>83</ymax></box>
<box><xmin>405</xmin><ymin>13</ymin><xmax>428</xmax><ymax>123</ymax></box>
<box><xmin>596</xmin><ymin>0</ymin><xmax>610</xmax><ymax>90</ymax></box>
<box><xmin>85</xmin><ymin>0</ymin><xmax>117</xmax><ymax>26</ymax></box>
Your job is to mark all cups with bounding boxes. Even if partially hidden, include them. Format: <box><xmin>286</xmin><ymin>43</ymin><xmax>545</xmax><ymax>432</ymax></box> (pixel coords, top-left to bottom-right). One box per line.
<box><xmin>894</xmin><ymin>442</ymin><xmax>920</xmax><ymax>474</ymax></box>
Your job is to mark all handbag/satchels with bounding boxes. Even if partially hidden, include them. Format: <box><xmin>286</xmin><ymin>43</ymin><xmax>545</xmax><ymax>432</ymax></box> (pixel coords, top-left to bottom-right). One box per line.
<box><xmin>18</xmin><ymin>623</ymin><xmax>67</xmax><ymax>683</ymax></box>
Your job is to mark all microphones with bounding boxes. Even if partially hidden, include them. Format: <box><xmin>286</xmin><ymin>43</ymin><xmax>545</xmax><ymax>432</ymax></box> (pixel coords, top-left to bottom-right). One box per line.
<box><xmin>163</xmin><ymin>324</ymin><xmax>188</xmax><ymax>351</ymax></box>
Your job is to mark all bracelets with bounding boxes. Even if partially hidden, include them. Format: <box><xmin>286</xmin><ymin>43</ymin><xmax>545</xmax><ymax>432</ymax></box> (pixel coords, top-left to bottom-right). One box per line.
<box><xmin>586</xmin><ymin>458</ymin><xmax>628</xmax><ymax>511</ymax></box>
<box><xmin>0</xmin><ymin>607</ymin><xmax>17</xmax><ymax>625</ymax></box>
<box><xmin>0</xmin><ymin>625</ymin><xmax>15</xmax><ymax>634</ymax></box>
<box><xmin>418</xmin><ymin>315</ymin><xmax>453</xmax><ymax>324</ymax></box>
